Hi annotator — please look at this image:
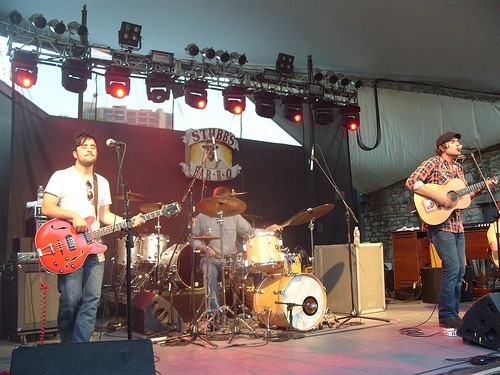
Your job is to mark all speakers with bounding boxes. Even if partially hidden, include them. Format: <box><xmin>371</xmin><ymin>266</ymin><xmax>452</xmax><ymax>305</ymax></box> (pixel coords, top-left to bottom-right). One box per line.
<box><xmin>457</xmin><ymin>291</ymin><xmax>500</xmax><ymax>351</ymax></box>
<box><xmin>418</xmin><ymin>267</ymin><xmax>474</xmax><ymax>303</ymax></box>
<box><xmin>1</xmin><ymin>264</ymin><xmax>61</xmax><ymax>332</ymax></box>
<box><xmin>130</xmin><ymin>290</ymin><xmax>185</xmax><ymax>334</ymax></box>
<box><xmin>10</xmin><ymin>337</ymin><xmax>156</xmax><ymax>375</ymax></box>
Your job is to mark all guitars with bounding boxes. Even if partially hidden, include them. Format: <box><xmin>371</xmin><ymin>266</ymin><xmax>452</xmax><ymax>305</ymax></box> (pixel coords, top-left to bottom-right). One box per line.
<box><xmin>34</xmin><ymin>200</ymin><xmax>183</xmax><ymax>275</ymax></box>
<box><xmin>410</xmin><ymin>176</ymin><xmax>500</xmax><ymax>225</ymax></box>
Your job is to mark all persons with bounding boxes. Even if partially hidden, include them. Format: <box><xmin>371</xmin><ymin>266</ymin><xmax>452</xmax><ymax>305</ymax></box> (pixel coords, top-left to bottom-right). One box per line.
<box><xmin>41</xmin><ymin>133</ymin><xmax>145</xmax><ymax>343</ymax></box>
<box><xmin>190</xmin><ymin>187</ymin><xmax>283</xmax><ymax>331</ymax></box>
<box><xmin>486</xmin><ymin>202</ymin><xmax>500</xmax><ymax>268</ymax></box>
<box><xmin>405</xmin><ymin>131</ymin><xmax>496</xmax><ymax>328</ymax></box>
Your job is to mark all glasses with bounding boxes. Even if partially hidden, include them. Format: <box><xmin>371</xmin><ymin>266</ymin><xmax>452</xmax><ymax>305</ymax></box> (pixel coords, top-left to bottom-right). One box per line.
<box><xmin>85</xmin><ymin>181</ymin><xmax>93</xmax><ymax>200</ymax></box>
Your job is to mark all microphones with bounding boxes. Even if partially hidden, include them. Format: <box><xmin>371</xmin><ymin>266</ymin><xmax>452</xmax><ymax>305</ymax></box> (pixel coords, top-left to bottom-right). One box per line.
<box><xmin>211</xmin><ymin>137</ymin><xmax>218</xmax><ymax>161</ymax></box>
<box><xmin>458</xmin><ymin>145</ymin><xmax>478</xmax><ymax>150</ymax></box>
<box><xmin>106</xmin><ymin>139</ymin><xmax>126</xmax><ymax>147</ymax></box>
<box><xmin>307</xmin><ymin>303</ymin><xmax>318</xmax><ymax>311</ymax></box>
<box><xmin>310</xmin><ymin>145</ymin><xmax>314</xmax><ymax>170</ymax></box>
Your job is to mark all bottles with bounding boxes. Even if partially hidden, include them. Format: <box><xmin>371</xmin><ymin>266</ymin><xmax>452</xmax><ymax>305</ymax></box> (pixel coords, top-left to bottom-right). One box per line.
<box><xmin>37</xmin><ymin>186</ymin><xmax>43</xmax><ymax>206</ymax></box>
<box><xmin>353</xmin><ymin>227</ymin><xmax>360</xmax><ymax>245</ymax></box>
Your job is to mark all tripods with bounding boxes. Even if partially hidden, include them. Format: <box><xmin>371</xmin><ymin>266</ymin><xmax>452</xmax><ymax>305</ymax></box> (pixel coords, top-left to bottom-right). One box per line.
<box><xmin>152</xmin><ymin>147</ymin><xmax>266</xmax><ymax>347</ymax></box>
<box><xmin>314</xmin><ymin>157</ymin><xmax>390</xmax><ymax>329</ymax></box>
<box><xmin>138</xmin><ymin>217</ymin><xmax>179</xmax><ymax>293</ymax></box>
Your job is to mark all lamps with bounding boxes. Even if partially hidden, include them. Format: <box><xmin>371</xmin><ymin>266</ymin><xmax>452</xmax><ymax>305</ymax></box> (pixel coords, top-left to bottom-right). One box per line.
<box><xmin>4</xmin><ymin>9</ymin><xmax>363</xmax><ymax>131</ymax></box>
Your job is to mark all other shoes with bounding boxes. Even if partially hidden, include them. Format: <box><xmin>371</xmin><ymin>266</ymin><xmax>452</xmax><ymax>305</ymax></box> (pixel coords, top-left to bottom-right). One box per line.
<box><xmin>439</xmin><ymin>316</ymin><xmax>462</xmax><ymax>328</ymax></box>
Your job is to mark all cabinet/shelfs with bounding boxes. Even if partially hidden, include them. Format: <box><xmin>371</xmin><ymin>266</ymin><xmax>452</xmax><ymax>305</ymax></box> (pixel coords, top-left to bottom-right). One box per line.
<box><xmin>390</xmin><ymin>228</ymin><xmax>490</xmax><ymax>298</ymax></box>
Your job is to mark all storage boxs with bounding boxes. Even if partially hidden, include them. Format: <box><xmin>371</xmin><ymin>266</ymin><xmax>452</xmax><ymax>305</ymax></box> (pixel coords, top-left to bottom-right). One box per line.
<box><xmin>314</xmin><ymin>242</ymin><xmax>386</xmax><ymax>316</ymax></box>
<box><xmin>18</xmin><ymin>261</ymin><xmax>57</xmax><ymax>333</ymax></box>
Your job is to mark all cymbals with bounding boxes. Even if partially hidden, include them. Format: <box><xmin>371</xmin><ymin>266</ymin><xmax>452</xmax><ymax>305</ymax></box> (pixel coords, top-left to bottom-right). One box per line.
<box><xmin>289</xmin><ymin>203</ymin><xmax>336</xmax><ymax>227</ymax></box>
<box><xmin>138</xmin><ymin>203</ymin><xmax>163</xmax><ymax>213</ymax></box>
<box><xmin>197</xmin><ymin>196</ymin><xmax>247</xmax><ymax>218</ymax></box>
<box><xmin>231</xmin><ymin>191</ymin><xmax>248</xmax><ymax>197</ymax></box>
<box><xmin>111</xmin><ymin>191</ymin><xmax>144</xmax><ymax>201</ymax></box>
<box><xmin>193</xmin><ymin>236</ymin><xmax>222</xmax><ymax>242</ymax></box>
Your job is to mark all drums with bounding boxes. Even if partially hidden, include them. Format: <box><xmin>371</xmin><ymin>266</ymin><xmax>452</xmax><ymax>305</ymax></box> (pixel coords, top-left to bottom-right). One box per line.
<box><xmin>160</xmin><ymin>242</ymin><xmax>205</xmax><ymax>290</ymax></box>
<box><xmin>241</xmin><ymin>231</ymin><xmax>285</xmax><ymax>273</ymax></box>
<box><xmin>115</xmin><ymin>236</ymin><xmax>140</xmax><ymax>269</ymax></box>
<box><xmin>267</xmin><ymin>253</ymin><xmax>301</xmax><ymax>276</ymax></box>
<box><xmin>137</xmin><ymin>233</ymin><xmax>171</xmax><ymax>264</ymax></box>
<box><xmin>252</xmin><ymin>272</ymin><xmax>328</xmax><ymax>331</ymax></box>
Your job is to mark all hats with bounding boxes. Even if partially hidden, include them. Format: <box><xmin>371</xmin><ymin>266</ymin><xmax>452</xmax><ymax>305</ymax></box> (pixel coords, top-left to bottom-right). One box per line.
<box><xmin>436</xmin><ymin>131</ymin><xmax>461</xmax><ymax>147</ymax></box>
